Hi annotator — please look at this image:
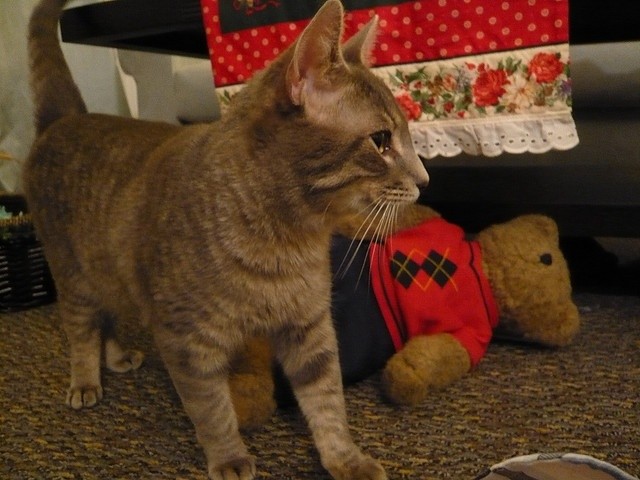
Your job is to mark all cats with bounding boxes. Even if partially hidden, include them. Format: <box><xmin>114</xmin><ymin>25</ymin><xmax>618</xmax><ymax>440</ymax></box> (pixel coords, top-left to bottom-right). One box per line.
<box><xmin>20</xmin><ymin>1</ymin><xmax>430</xmax><ymax>480</ymax></box>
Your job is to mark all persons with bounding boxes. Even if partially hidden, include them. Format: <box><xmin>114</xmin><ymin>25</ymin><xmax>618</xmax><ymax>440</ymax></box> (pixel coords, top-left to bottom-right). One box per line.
<box><xmin>228</xmin><ymin>203</ymin><xmax>580</xmax><ymax>432</ymax></box>
